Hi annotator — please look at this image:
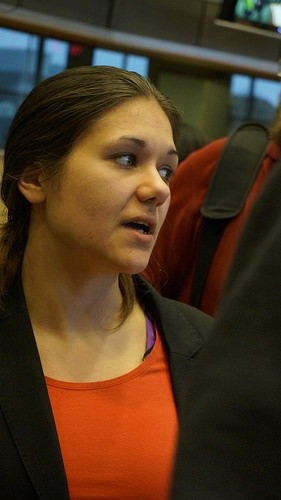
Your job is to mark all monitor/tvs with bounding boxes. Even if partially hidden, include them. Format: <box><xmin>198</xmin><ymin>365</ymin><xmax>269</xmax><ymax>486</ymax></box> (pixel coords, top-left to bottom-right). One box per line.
<box><xmin>215</xmin><ymin>0</ymin><xmax>281</xmax><ymax>40</ymax></box>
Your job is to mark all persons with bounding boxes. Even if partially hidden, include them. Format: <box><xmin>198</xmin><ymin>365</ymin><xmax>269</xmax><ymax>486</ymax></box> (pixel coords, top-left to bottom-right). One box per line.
<box><xmin>0</xmin><ymin>61</ymin><xmax>224</xmax><ymax>500</ymax></box>
<box><xmin>202</xmin><ymin>164</ymin><xmax>280</xmax><ymax>500</ymax></box>
<box><xmin>139</xmin><ymin>94</ymin><xmax>281</xmax><ymax>316</ymax></box>
<box><xmin>170</xmin><ymin>123</ymin><xmax>212</xmax><ymax>159</ymax></box>
